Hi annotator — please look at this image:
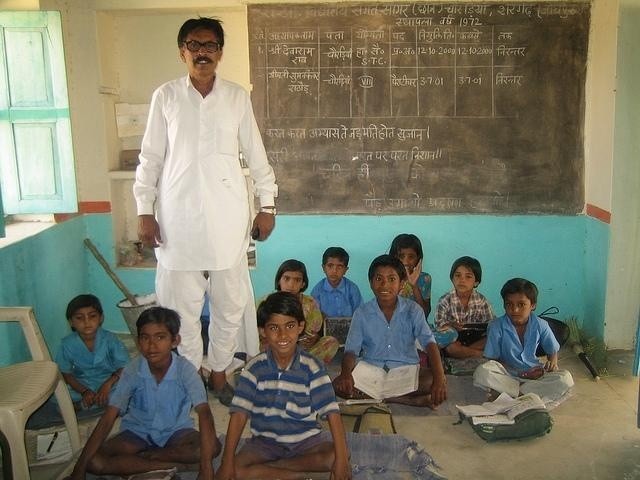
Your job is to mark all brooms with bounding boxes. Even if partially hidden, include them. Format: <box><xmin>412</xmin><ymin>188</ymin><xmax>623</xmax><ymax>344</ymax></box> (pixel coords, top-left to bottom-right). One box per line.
<box><xmin>561</xmin><ymin>316</ymin><xmax>600</xmax><ymax>382</ymax></box>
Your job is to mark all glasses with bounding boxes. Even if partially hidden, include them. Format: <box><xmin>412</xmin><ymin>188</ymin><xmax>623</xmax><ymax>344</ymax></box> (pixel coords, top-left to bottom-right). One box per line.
<box><xmin>182</xmin><ymin>39</ymin><xmax>220</xmax><ymax>53</ymax></box>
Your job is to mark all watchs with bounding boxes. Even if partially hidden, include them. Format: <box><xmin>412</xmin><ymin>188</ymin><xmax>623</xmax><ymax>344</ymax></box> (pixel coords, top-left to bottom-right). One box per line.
<box><xmin>258</xmin><ymin>206</ymin><xmax>277</xmax><ymax>216</ymax></box>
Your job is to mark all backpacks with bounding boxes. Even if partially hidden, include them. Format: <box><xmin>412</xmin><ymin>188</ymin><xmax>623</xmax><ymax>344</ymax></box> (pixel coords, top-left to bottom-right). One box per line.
<box><xmin>458</xmin><ymin>408</ymin><xmax>553</xmax><ymax>444</ymax></box>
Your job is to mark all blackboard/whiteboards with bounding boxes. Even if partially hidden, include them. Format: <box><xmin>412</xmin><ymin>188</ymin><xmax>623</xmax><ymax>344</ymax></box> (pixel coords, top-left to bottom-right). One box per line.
<box><xmin>247</xmin><ymin>3</ymin><xmax>591</xmax><ymax>215</ymax></box>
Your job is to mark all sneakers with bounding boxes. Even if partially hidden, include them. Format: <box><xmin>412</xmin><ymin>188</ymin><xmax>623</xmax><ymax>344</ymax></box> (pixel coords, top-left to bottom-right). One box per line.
<box><xmin>200</xmin><ymin>366</ymin><xmax>208</xmax><ymax>390</ymax></box>
<box><xmin>208</xmin><ymin>370</ymin><xmax>237</xmax><ymax>408</ymax></box>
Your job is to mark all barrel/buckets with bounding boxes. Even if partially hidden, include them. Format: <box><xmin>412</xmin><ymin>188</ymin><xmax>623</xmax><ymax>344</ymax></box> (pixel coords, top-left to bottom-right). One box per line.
<box><xmin>117</xmin><ymin>294</ymin><xmax>156</xmax><ymax>352</ymax></box>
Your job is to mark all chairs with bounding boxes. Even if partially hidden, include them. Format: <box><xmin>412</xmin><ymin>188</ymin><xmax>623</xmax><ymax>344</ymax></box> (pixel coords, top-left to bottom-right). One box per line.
<box><xmin>0</xmin><ymin>306</ymin><xmax>83</xmax><ymax>480</ymax></box>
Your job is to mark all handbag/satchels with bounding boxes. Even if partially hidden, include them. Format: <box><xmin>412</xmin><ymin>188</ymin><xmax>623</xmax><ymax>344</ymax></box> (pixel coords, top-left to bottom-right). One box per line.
<box><xmin>457</xmin><ymin>322</ymin><xmax>490</xmax><ymax>347</ymax></box>
<box><xmin>535</xmin><ymin>315</ymin><xmax>570</xmax><ymax>358</ymax></box>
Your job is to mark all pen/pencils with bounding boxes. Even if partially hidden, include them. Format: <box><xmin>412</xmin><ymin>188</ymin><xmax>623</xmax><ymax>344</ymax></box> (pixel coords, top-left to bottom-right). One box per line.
<box><xmin>46</xmin><ymin>431</ymin><xmax>58</xmax><ymax>454</ymax></box>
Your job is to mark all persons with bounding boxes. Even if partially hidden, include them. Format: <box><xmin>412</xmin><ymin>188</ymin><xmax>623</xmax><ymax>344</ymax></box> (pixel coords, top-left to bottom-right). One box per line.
<box><xmin>200</xmin><ymin>293</ymin><xmax>214</xmax><ymax>357</ymax></box>
<box><xmin>131</xmin><ymin>16</ymin><xmax>282</xmax><ymax>375</ymax></box>
<box><xmin>470</xmin><ymin>275</ymin><xmax>576</xmax><ymax>407</ymax></box>
<box><xmin>309</xmin><ymin>246</ymin><xmax>363</xmax><ymax>318</ymax></box>
<box><xmin>50</xmin><ymin>292</ymin><xmax>130</xmax><ymax>417</ymax></box>
<box><xmin>256</xmin><ymin>259</ymin><xmax>340</xmax><ymax>368</ymax></box>
<box><xmin>330</xmin><ymin>253</ymin><xmax>448</xmax><ymax>411</ymax></box>
<box><xmin>214</xmin><ymin>289</ymin><xmax>353</xmax><ymax>480</ymax></box>
<box><xmin>433</xmin><ymin>254</ymin><xmax>497</xmax><ymax>361</ymax></box>
<box><xmin>388</xmin><ymin>233</ymin><xmax>458</xmax><ymax>351</ymax></box>
<box><xmin>61</xmin><ymin>305</ymin><xmax>222</xmax><ymax>480</ymax></box>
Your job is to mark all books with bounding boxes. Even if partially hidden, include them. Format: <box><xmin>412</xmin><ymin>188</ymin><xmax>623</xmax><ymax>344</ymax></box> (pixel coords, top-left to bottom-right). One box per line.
<box><xmin>124</xmin><ymin>466</ymin><xmax>178</xmax><ymax>480</ymax></box>
<box><xmin>456</xmin><ymin>391</ymin><xmax>523</xmax><ymax>417</ymax></box>
<box><xmin>471</xmin><ymin>392</ymin><xmax>546</xmax><ymax>426</ymax></box>
<box><xmin>350</xmin><ymin>358</ymin><xmax>421</xmax><ymax>401</ymax></box>
